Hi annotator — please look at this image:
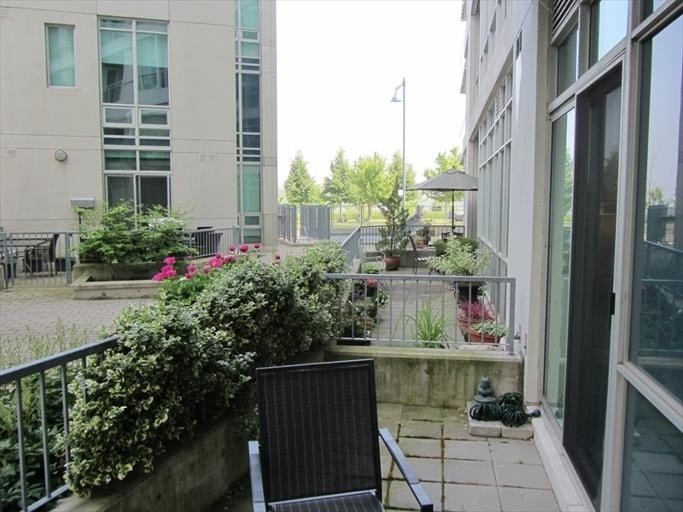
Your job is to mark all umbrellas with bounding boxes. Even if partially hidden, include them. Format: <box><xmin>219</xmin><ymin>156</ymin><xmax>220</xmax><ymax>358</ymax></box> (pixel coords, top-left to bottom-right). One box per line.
<box><xmin>405</xmin><ymin>167</ymin><xmax>477</xmax><ymax>233</ymax></box>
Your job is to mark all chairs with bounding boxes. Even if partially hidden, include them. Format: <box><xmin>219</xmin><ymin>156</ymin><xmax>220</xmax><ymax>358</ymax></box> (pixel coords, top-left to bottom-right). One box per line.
<box><xmin>247</xmin><ymin>356</ymin><xmax>434</xmax><ymax>512</ymax></box>
<box><xmin>23</xmin><ymin>233</ymin><xmax>59</xmax><ymax>278</ymax></box>
<box><xmin>408</xmin><ymin>234</ymin><xmax>432</xmax><ymax>275</ymax></box>
<box><xmin>182</xmin><ymin>226</ymin><xmax>224</xmax><ymax>254</ymax></box>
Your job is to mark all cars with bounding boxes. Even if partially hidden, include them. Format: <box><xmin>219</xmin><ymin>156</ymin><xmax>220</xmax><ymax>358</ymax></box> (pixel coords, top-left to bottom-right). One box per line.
<box><xmin>447</xmin><ymin>208</ymin><xmax>464</xmax><ymax>222</ymax></box>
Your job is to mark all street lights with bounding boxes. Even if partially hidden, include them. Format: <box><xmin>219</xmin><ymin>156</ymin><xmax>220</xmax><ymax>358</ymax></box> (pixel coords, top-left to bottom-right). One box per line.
<box><xmin>387</xmin><ymin>74</ymin><xmax>407</xmax><ymax>225</ymax></box>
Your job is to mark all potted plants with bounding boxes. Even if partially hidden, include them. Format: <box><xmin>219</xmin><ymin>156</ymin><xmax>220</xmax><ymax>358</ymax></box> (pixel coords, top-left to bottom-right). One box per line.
<box><xmin>337</xmin><ymin>297</ymin><xmax>377</xmax><ymax>345</ymax></box>
<box><xmin>426</xmin><ymin>226</ymin><xmax>507</xmax><ymax>346</ymax></box>
<box><xmin>374</xmin><ymin>172</ymin><xmax>412</xmax><ymax>271</ymax></box>
